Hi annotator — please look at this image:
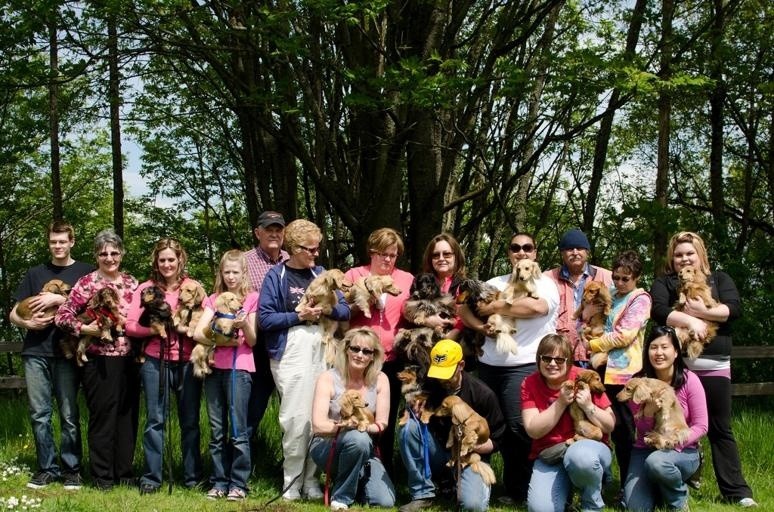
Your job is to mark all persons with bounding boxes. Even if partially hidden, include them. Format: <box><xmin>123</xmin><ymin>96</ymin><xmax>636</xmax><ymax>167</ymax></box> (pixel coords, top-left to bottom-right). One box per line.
<box><xmin>542</xmin><ymin>230</ymin><xmax>617</xmax><ymax>494</ymax></box>
<box><xmin>257</xmin><ymin>219</ymin><xmax>353</xmax><ymax>502</ymax></box>
<box><xmin>520</xmin><ymin>334</ymin><xmax>616</xmax><ymax>512</ymax></box>
<box><xmin>192</xmin><ymin>249</ymin><xmax>260</xmax><ymax>501</ymax></box>
<box><xmin>309</xmin><ymin>328</ymin><xmax>396</xmax><ymax>511</ymax></box>
<box><xmin>459</xmin><ymin>232</ymin><xmax>560</xmax><ymax>506</ymax></box>
<box><xmin>241</xmin><ymin>211</ymin><xmax>290</xmax><ymax>455</ymax></box>
<box><xmin>650</xmin><ymin>232</ymin><xmax>759</xmax><ymax>506</ymax></box>
<box><xmin>581</xmin><ymin>250</ymin><xmax>654</xmax><ymax>502</ymax></box>
<box><xmin>341</xmin><ymin>228</ymin><xmax>415</xmax><ymax>480</ymax></box>
<box><xmin>9</xmin><ymin>221</ymin><xmax>97</xmax><ymax>490</ymax></box>
<box><xmin>54</xmin><ymin>231</ymin><xmax>139</xmax><ymax>491</ymax></box>
<box><xmin>124</xmin><ymin>239</ymin><xmax>209</xmax><ymax>494</ymax></box>
<box><xmin>399</xmin><ymin>232</ymin><xmax>478</xmax><ymax>476</ymax></box>
<box><xmin>398</xmin><ymin>340</ymin><xmax>506</xmax><ymax>512</ymax></box>
<box><xmin>621</xmin><ymin>327</ymin><xmax>708</xmax><ymax>512</ymax></box>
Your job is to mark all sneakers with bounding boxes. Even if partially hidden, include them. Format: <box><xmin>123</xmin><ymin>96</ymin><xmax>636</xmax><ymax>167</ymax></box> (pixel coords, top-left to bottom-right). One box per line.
<box><xmin>228</xmin><ymin>486</ymin><xmax>247</xmax><ymax>501</ymax></box>
<box><xmin>740</xmin><ymin>497</ymin><xmax>758</xmax><ymax>508</ymax></box>
<box><xmin>283</xmin><ymin>488</ymin><xmax>325</xmax><ymax>502</ymax></box>
<box><xmin>139</xmin><ymin>482</ymin><xmax>158</xmax><ymax>493</ymax></box>
<box><xmin>206</xmin><ymin>487</ymin><xmax>225</xmax><ymax>501</ymax></box>
<box><xmin>117</xmin><ymin>474</ymin><xmax>139</xmax><ymax>487</ymax></box>
<box><xmin>329</xmin><ymin>496</ymin><xmax>349</xmax><ymax>512</ymax></box>
<box><xmin>91</xmin><ymin>478</ymin><xmax>114</xmax><ymax>490</ymax></box>
<box><xmin>64</xmin><ymin>470</ymin><xmax>83</xmax><ymax>490</ymax></box>
<box><xmin>398</xmin><ymin>500</ymin><xmax>433</xmax><ymax>512</ymax></box>
<box><xmin>28</xmin><ymin>468</ymin><xmax>61</xmax><ymax>490</ymax></box>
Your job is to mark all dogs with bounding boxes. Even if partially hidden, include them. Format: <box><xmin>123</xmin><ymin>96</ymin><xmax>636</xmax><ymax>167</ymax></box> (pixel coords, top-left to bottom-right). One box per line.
<box><xmin>395</xmin><ymin>364</ymin><xmax>452</xmax><ymax>453</ymax></box>
<box><xmin>559</xmin><ymin>368</ymin><xmax>607</xmax><ymax>447</ymax></box>
<box><xmin>339</xmin><ymin>273</ymin><xmax>403</xmax><ymax>338</ymax></box>
<box><xmin>672</xmin><ymin>264</ymin><xmax>720</xmax><ymax>362</ymax></box>
<box><xmin>16</xmin><ymin>279</ymin><xmax>72</xmax><ymax>320</ymax></box>
<box><xmin>393</xmin><ymin>258</ymin><xmax>542</xmax><ymax>362</ymax></box>
<box><xmin>57</xmin><ymin>286</ymin><xmax>124</xmax><ymax>368</ymax></box>
<box><xmin>434</xmin><ymin>394</ymin><xmax>497</xmax><ymax>487</ymax></box>
<box><xmin>126</xmin><ymin>277</ymin><xmax>245</xmax><ymax>377</ymax></box>
<box><xmin>615</xmin><ymin>375</ymin><xmax>691</xmax><ymax>451</ymax></box>
<box><xmin>294</xmin><ymin>268</ymin><xmax>354</xmax><ymax>372</ymax></box>
<box><xmin>335</xmin><ymin>389</ymin><xmax>375</xmax><ymax>432</ymax></box>
<box><xmin>570</xmin><ymin>280</ymin><xmax>613</xmax><ymax>371</ymax></box>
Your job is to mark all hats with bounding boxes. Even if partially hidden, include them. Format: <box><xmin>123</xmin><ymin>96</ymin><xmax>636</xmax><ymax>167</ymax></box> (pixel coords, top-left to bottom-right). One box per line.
<box><xmin>427</xmin><ymin>339</ymin><xmax>464</xmax><ymax>381</ymax></box>
<box><xmin>256</xmin><ymin>210</ymin><xmax>286</xmax><ymax>228</ymax></box>
<box><xmin>560</xmin><ymin>228</ymin><xmax>590</xmax><ymax>252</ymax></box>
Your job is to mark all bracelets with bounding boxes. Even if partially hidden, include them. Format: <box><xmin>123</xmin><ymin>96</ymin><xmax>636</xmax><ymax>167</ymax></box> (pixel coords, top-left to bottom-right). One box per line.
<box><xmin>578</xmin><ymin>313</ymin><xmax>588</xmax><ymax>323</ymax></box>
<box><xmin>587</xmin><ymin>405</ymin><xmax>597</xmax><ymax>418</ymax></box>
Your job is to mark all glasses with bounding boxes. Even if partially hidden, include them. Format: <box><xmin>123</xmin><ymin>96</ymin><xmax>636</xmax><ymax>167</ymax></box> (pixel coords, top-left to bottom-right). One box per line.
<box><xmin>372</xmin><ymin>251</ymin><xmax>398</xmax><ymax>258</ymax></box>
<box><xmin>97</xmin><ymin>252</ymin><xmax>122</xmax><ymax>258</ymax></box>
<box><xmin>537</xmin><ymin>353</ymin><xmax>568</xmax><ymax>365</ymax></box>
<box><xmin>347</xmin><ymin>344</ymin><xmax>376</xmax><ymax>354</ymax></box>
<box><xmin>428</xmin><ymin>252</ymin><xmax>455</xmax><ymax>259</ymax></box>
<box><xmin>509</xmin><ymin>241</ymin><xmax>536</xmax><ymax>253</ymax></box>
<box><xmin>612</xmin><ymin>274</ymin><xmax>632</xmax><ymax>282</ymax></box>
<box><xmin>297</xmin><ymin>244</ymin><xmax>321</xmax><ymax>253</ymax></box>
